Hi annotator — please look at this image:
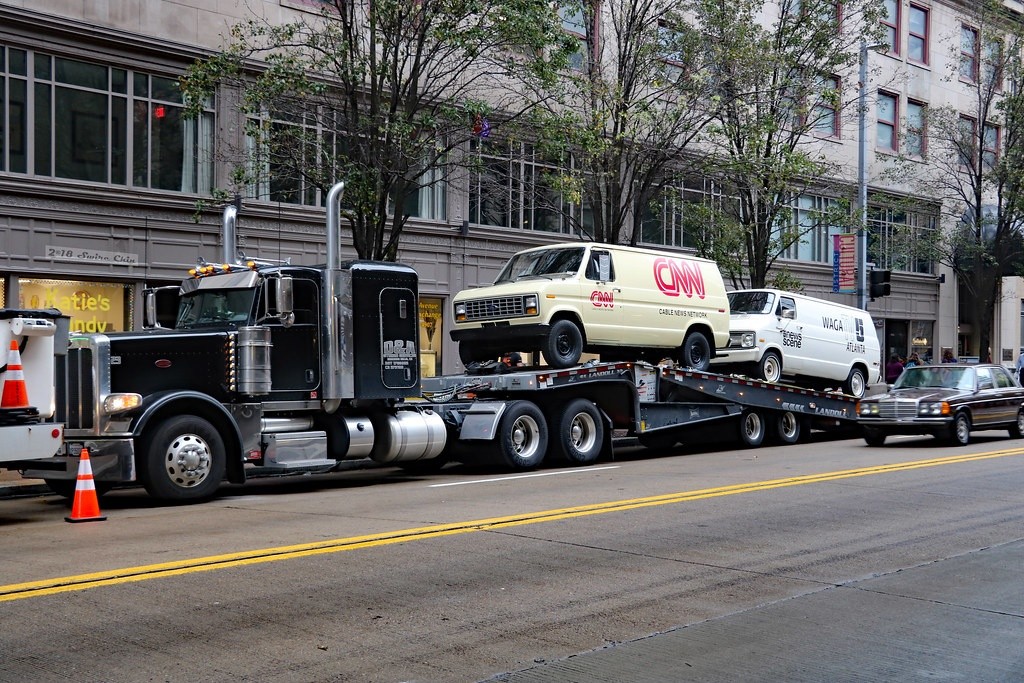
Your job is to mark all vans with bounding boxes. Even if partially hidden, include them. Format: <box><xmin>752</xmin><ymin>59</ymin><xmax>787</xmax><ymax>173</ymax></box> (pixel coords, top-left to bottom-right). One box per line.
<box><xmin>706</xmin><ymin>288</ymin><xmax>881</xmax><ymax>400</ymax></box>
<box><xmin>450</xmin><ymin>244</ymin><xmax>734</xmax><ymax>371</ymax></box>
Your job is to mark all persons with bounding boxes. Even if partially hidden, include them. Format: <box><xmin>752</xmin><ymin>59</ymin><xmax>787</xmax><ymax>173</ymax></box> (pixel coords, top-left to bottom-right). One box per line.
<box><xmin>1016</xmin><ymin>347</ymin><xmax>1024</xmax><ymax>387</ymax></box>
<box><xmin>886</xmin><ymin>350</ymin><xmax>957</xmax><ymax>385</ymax></box>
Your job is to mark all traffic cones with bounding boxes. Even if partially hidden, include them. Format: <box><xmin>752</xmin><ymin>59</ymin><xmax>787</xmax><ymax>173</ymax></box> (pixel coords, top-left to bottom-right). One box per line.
<box><xmin>65</xmin><ymin>448</ymin><xmax>108</xmax><ymax>523</ymax></box>
<box><xmin>0</xmin><ymin>339</ymin><xmax>39</xmax><ymax>411</ymax></box>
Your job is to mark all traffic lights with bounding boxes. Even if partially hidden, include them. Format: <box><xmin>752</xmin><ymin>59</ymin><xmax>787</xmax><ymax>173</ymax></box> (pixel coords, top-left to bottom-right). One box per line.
<box><xmin>869</xmin><ymin>270</ymin><xmax>891</xmax><ymax>299</ymax></box>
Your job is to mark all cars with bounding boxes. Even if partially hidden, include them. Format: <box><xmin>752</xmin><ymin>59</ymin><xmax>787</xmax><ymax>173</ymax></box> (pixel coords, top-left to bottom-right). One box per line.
<box><xmin>855</xmin><ymin>363</ymin><xmax>1024</xmax><ymax>447</ymax></box>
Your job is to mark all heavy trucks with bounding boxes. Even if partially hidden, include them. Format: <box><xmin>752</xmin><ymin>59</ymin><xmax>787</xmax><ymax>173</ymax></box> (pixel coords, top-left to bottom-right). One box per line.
<box><xmin>0</xmin><ymin>175</ymin><xmax>886</xmax><ymax>508</ymax></box>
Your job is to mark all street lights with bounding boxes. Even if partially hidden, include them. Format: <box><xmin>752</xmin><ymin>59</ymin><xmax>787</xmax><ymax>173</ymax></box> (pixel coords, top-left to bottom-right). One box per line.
<box><xmin>858</xmin><ymin>40</ymin><xmax>891</xmax><ymax>310</ymax></box>
